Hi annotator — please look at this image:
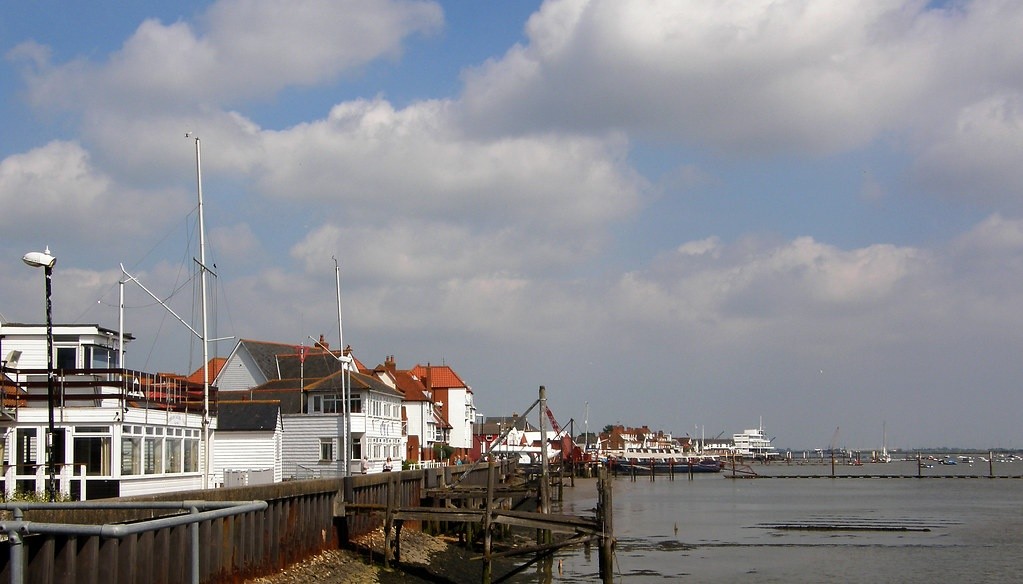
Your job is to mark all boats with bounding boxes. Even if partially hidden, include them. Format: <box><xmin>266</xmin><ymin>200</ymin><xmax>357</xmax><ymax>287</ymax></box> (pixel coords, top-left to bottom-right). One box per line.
<box><xmin>600</xmin><ymin>452</ymin><xmax>727</xmax><ymax>475</ymax></box>
<box><xmin>844</xmin><ymin>421</ymin><xmax>1023</xmax><ymax>469</ymax></box>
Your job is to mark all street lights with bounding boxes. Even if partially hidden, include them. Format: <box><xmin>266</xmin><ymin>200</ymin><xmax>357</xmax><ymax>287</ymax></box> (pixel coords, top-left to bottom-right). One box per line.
<box><xmin>339</xmin><ymin>354</ymin><xmax>354</xmax><ymax>477</ymax></box>
<box><xmin>434</xmin><ymin>401</ymin><xmax>443</xmax><ymax>468</ymax></box>
<box><xmin>21</xmin><ymin>245</ymin><xmax>59</xmax><ymax>503</ymax></box>
<box><xmin>476</xmin><ymin>413</ymin><xmax>484</xmax><ymax>457</ymax></box>
<box><xmin>496</xmin><ymin>422</ymin><xmax>501</xmax><ymax>459</ymax></box>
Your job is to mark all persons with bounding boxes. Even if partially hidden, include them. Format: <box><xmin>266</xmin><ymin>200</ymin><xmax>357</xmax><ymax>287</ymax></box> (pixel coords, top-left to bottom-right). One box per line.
<box><xmin>450</xmin><ymin>451</ymin><xmax>511</xmax><ymax>466</ymax></box>
<box><xmin>383</xmin><ymin>456</ymin><xmax>393</xmax><ymax>473</ymax></box>
<box><xmin>361</xmin><ymin>455</ymin><xmax>370</xmax><ymax>474</ymax></box>
